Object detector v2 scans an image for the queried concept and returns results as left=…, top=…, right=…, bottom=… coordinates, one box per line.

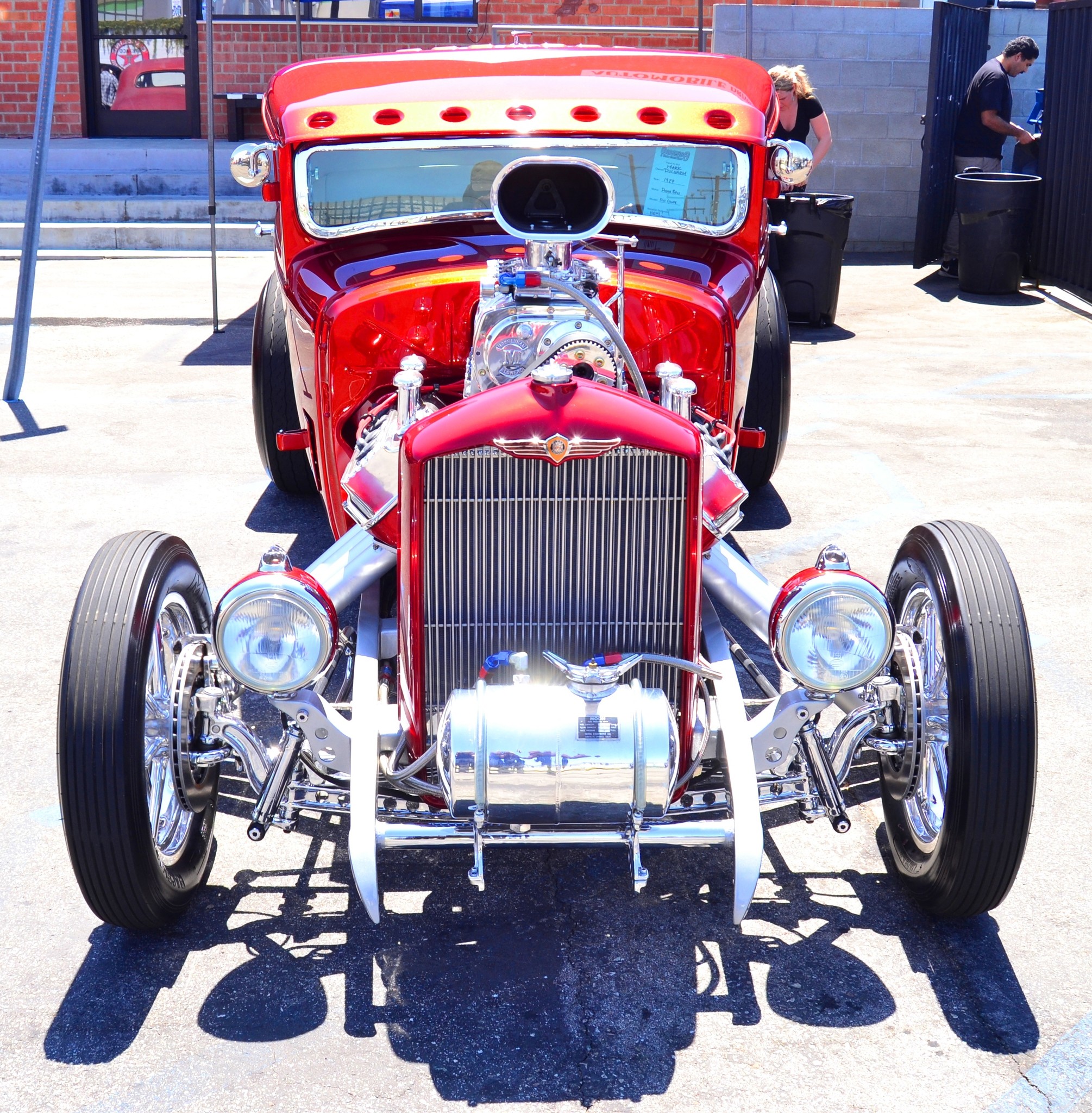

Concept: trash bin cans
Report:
left=954, top=166, right=1042, bottom=296
left=767, top=191, right=853, bottom=330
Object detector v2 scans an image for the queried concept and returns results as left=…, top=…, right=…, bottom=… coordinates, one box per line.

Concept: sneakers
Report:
left=938, top=263, right=959, bottom=278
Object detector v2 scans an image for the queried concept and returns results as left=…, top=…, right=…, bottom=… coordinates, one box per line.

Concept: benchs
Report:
left=213, top=93, right=264, bottom=143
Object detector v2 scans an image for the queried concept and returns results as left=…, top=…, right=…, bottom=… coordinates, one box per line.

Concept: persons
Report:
left=768, top=63, right=832, bottom=194
left=938, top=36, right=1039, bottom=279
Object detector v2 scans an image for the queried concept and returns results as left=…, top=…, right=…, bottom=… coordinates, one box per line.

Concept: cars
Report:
left=56, top=25, right=1039, bottom=938
